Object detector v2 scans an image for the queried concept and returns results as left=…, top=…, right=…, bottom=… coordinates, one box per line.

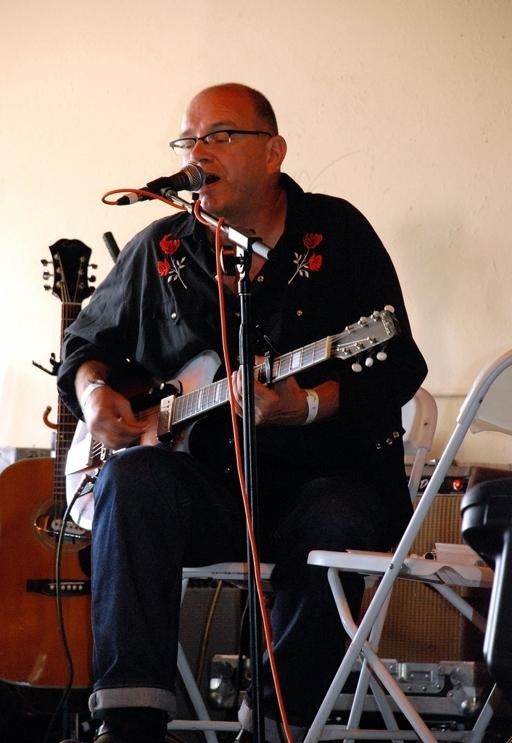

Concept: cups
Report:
left=169, top=129, right=272, bottom=155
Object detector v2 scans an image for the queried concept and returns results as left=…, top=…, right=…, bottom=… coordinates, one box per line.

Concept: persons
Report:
left=59, top=83, right=426, bottom=742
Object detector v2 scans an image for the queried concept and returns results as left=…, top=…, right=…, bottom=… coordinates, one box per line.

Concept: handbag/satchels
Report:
left=304, top=389, right=318, bottom=424
left=79, top=379, right=106, bottom=411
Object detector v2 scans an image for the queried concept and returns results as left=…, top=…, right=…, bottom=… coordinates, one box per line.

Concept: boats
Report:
left=358, top=464, right=472, bottom=664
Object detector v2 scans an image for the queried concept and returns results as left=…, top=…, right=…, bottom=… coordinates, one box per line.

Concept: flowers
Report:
left=117, top=164, right=204, bottom=206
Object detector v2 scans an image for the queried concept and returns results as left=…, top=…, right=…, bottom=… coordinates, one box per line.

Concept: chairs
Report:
left=165, top=352, right=512, bottom=741
